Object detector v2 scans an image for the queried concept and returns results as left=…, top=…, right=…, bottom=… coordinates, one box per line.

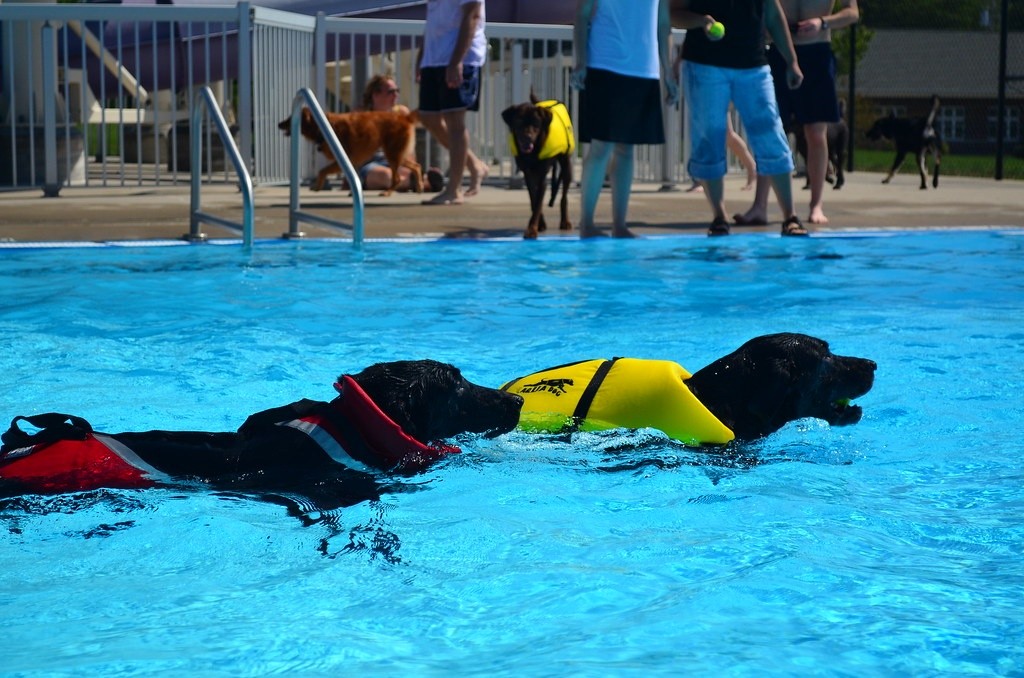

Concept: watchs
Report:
left=818, top=16, right=828, bottom=31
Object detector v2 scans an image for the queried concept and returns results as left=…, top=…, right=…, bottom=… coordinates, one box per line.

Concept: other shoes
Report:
left=410, top=167, right=444, bottom=192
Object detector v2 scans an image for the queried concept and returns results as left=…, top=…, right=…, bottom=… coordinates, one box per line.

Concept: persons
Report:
left=667, top=1, right=808, bottom=237
left=412, top=1, right=492, bottom=206
left=567, top=1, right=681, bottom=240
left=731, top=1, right=860, bottom=225
left=347, top=72, right=445, bottom=194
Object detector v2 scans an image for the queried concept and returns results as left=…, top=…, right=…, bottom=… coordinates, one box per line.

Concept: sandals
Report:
left=780, top=216, right=809, bottom=237
left=709, top=217, right=730, bottom=236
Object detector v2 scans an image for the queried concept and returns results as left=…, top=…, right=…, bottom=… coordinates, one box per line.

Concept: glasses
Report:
left=385, top=87, right=400, bottom=94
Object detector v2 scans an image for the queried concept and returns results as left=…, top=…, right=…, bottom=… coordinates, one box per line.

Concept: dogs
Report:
left=278, top=107, right=429, bottom=197
left=781, top=98, right=850, bottom=190
left=501, top=83, right=575, bottom=240
left=679, top=332, right=877, bottom=446
left=96, top=359, right=525, bottom=491
left=866, top=92, right=944, bottom=190
